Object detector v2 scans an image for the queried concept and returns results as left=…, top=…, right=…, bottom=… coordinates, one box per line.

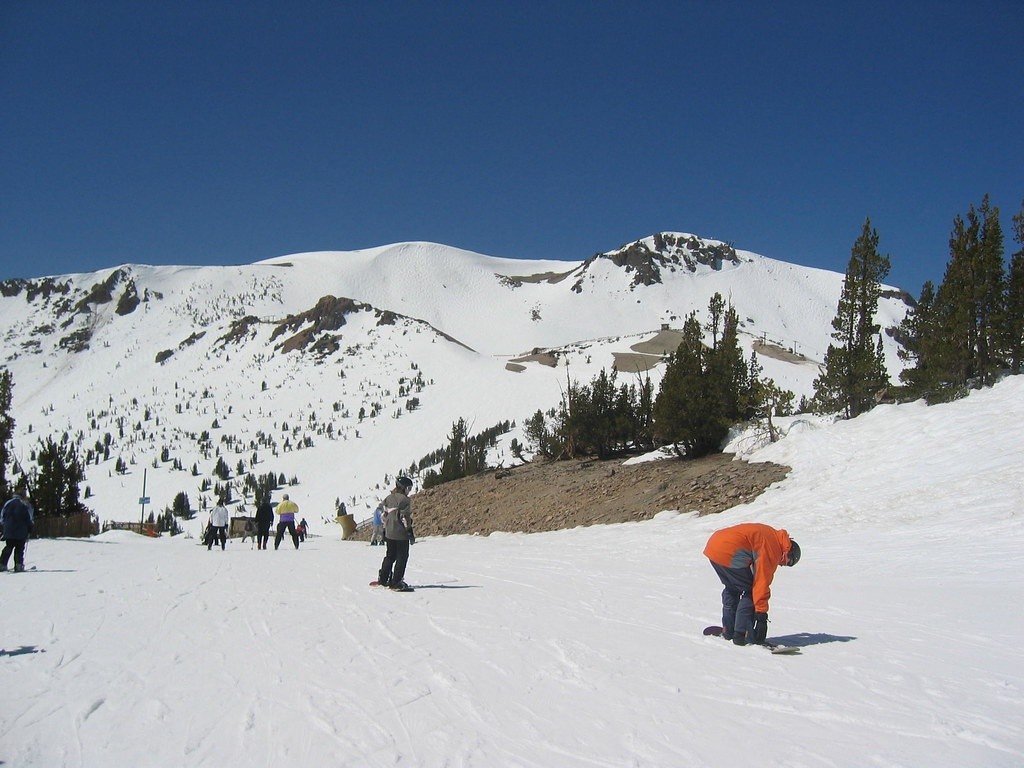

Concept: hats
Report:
left=283, top=494, right=289, bottom=499
left=787, top=540, right=801, bottom=567
left=396, top=477, right=413, bottom=490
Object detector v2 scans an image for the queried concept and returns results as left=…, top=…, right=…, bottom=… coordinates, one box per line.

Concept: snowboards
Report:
left=369, top=581, right=414, bottom=592
left=703, top=626, right=800, bottom=654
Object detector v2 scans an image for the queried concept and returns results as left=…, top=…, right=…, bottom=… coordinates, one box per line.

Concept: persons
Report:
left=257, top=497, right=274, bottom=550
left=378, top=477, right=415, bottom=589
left=703, top=523, right=801, bottom=645
left=208, top=499, right=228, bottom=550
left=370, top=504, right=385, bottom=545
left=0, top=486, right=34, bottom=572
left=201, top=509, right=309, bottom=546
left=274, top=494, right=299, bottom=550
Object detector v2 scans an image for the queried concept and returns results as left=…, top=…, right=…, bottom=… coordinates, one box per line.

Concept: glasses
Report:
left=406, top=486, right=412, bottom=490
left=787, top=559, right=791, bottom=566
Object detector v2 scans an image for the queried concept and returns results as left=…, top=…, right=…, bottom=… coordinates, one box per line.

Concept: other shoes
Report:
left=378, top=581, right=389, bottom=586
left=734, top=632, right=748, bottom=645
left=722, top=627, right=734, bottom=640
left=390, top=579, right=414, bottom=592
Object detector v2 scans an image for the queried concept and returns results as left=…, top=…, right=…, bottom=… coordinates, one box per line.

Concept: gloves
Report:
left=383, top=528, right=387, bottom=543
left=408, top=529, right=415, bottom=545
left=754, top=613, right=769, bottom=641
